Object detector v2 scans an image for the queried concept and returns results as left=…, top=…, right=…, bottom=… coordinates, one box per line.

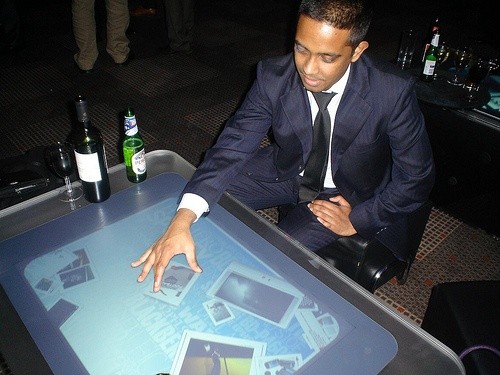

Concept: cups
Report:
left=472, top=57, right=485, bottom=72
left=462, top=79, right=480, bottom=104
left=397, top=28, right=418, bottom=64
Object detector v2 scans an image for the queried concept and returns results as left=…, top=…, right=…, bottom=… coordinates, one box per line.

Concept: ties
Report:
left=298, top=91, right=338, bottom=203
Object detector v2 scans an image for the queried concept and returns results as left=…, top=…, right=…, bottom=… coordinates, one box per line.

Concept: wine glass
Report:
left=48, top=141, right=83, bottom=202
left=447, top=47, right=473, bottom=85
left=433, top=42, right=450, bottom=80
left=487, top=58, right=499, bottom=75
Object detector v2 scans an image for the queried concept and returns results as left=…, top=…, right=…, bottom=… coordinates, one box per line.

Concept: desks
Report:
left=0, top=150, right=465, bottom=375
left=404, top=57, right=491, bottom=108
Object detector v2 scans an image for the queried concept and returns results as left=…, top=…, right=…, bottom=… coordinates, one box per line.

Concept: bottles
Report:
left=69, top=95, right=111, bottom=203
left=421, top=29, right=441, bottom=82
left=122, top=107, right=147, bottom=183
left=420, top=16, right=441, bottom=64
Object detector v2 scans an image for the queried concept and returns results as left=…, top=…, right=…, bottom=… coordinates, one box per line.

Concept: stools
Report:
left=278, top=202, right=434, bottom=295
left=421, top=280, right=500, bottom=375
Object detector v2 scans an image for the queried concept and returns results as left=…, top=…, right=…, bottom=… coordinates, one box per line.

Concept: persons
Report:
left=164, top=0, right=194, bottom=56
left=71, top=0, right=131, bottom=74
left=131, top=0, right=435, bottom=294
